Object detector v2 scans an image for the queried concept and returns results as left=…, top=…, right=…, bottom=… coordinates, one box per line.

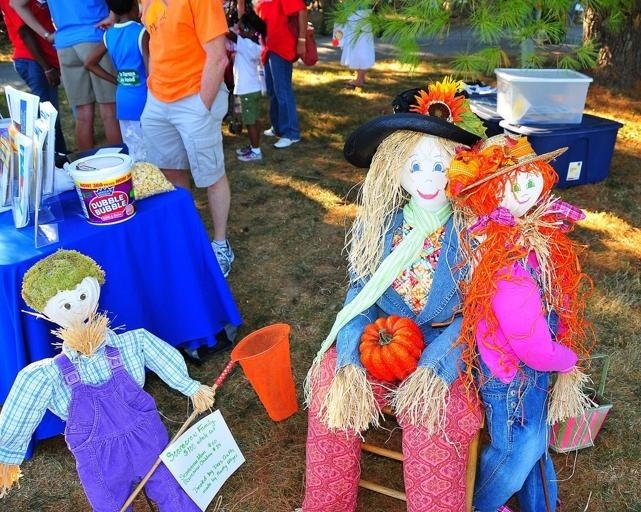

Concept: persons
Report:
left=300, top=85, right=483, bottom=512
left=1, top=248, right=215, bottom=512
left=446, top=132, right=577, bottom=512
left=0, top=0, right=375, bottom=278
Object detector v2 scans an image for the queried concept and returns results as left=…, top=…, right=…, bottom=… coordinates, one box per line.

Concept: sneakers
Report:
left=273, top=136, right=303, bottom=149
left=235, top=144, right=256, bottom=156
left=263, top=126, right=277, bottom=136
left=237, top=152, right=263, bottom=162
left=344, top=79, right=366, bottom=88
left=210, top=239, right=238, bottom=278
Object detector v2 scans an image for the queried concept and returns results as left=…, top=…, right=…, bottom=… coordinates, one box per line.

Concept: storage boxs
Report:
left=500, top=112, right=623, bottom=188
left=470, top=101, right=503, bottom=138
left=496, top=68, right=593, bottom=124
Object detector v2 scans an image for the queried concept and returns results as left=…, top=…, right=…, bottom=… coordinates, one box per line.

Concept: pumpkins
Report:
left=359, top=315, right=424, bottom=383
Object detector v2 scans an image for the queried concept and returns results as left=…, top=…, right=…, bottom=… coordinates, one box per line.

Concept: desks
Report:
left=0, top=147, right=243, bottom=460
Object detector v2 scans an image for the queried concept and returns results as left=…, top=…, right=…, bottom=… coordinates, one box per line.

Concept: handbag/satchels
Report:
left=286, top=12, right=319, bottom=66
